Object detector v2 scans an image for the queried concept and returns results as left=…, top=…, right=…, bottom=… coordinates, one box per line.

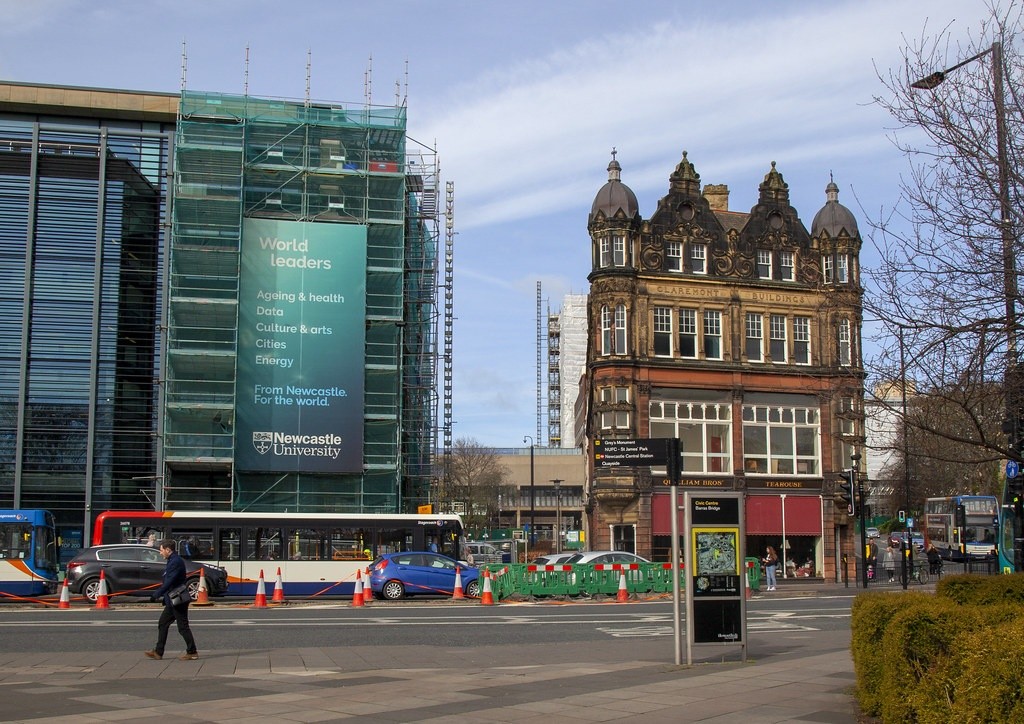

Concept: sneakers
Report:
left=769, top=586, right=776, bottom=591
left=767, top=586, right=770, bottom=591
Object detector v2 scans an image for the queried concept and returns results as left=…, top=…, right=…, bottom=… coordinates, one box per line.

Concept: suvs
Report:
left=65, top=543, right=228, bottom=604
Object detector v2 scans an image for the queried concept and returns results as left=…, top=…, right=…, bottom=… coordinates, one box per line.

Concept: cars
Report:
left=888, top=531, right=924, bottom=551
left=865, top=527, right=880, bottom=538
left=367, top=551, right=667, bottom=599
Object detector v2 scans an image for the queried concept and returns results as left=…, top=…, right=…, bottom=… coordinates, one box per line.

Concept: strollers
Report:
left=932, top=556, right=945, bottom=573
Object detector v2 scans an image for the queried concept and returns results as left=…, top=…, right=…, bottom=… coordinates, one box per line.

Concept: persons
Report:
left=883, top=546, right=895, bottom=582
left=912, top=542, right=921, bottom=578
left=143, top=534, right=157, bottom=562
left=762, top=546, right=777, bottom=591
left=146, top=540, right=199, bottom=660
left=927, top=543, right=939, bottom=574
left=758, top=556, right=814, bottom=577
left=429, top=557, right=449, bottom=568
left=867, top=538, right=878, bottom=581
left=430, top=537, right=449, bottom=555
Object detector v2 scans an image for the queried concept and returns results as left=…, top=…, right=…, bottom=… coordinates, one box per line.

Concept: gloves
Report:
left=150, top=597, right=155, bottom=602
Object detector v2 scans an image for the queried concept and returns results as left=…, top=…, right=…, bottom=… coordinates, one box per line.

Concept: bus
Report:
left=998, top=469, right=1014, bottom=574
left=923, top=495, right=1002, bottom=564
left=0, top=509, right=468, bottom=598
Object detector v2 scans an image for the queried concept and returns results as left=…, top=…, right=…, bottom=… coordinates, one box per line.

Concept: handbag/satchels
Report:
left=168, top=584, right=192, bottom=608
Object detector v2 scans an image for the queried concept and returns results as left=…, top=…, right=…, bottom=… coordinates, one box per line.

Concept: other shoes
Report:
left=888, top=579, right=891, bottom=583
left=145, top=647, right=162, bottom=660
left=891, top=577, right=895, bottom=582
left=178, top=651, right=199, bottom=660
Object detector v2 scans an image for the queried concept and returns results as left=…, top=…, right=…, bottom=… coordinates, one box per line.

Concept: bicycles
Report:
left=899, top=558, right=929, bottom=585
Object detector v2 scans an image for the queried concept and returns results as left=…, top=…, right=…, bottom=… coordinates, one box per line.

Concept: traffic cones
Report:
left=450, top=564, right=501, bottom=606
left=249, top=567, right=287, bottom=609
left=614, top=566, right=631, bottom=603
left=190, top=568, right=215, bottom=606
left=349, top=566, right=375, bottom=607
left=57, top=568, right=115, bottom=611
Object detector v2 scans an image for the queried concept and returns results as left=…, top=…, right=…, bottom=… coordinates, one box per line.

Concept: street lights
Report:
left=910, top=40, right=1024, bottom=572
left=550, top=479, right=565, bottom=553
left=523, top=436, right=534, bottom=551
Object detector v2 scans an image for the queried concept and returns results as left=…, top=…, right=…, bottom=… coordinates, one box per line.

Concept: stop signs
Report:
left=840, top=469, right=854, bottom=517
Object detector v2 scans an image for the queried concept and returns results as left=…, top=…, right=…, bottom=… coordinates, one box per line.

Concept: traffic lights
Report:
left=899, top=510, right=905, bottom=522
